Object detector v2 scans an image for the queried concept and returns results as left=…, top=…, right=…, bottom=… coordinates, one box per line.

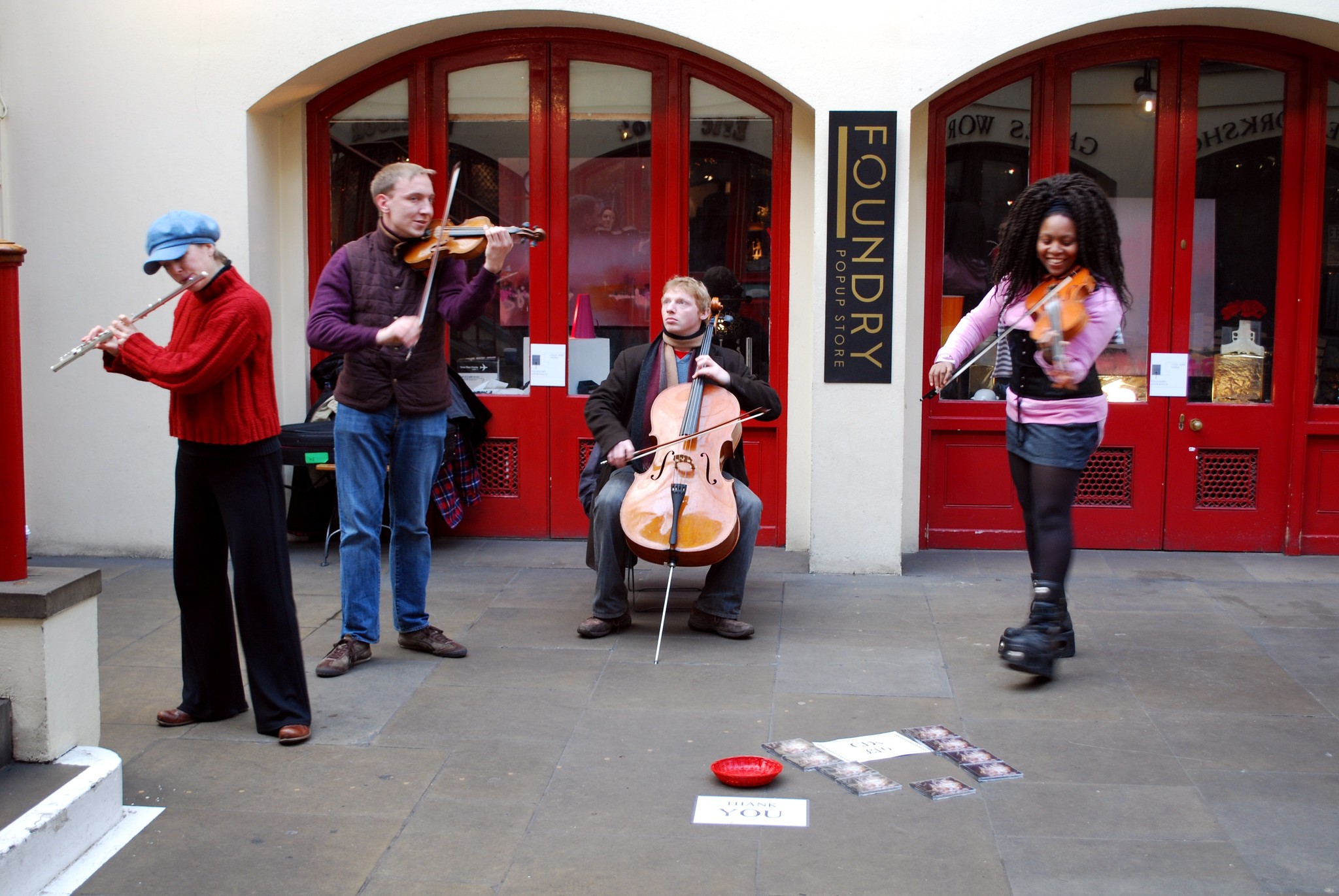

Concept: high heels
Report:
left=1005, top=579, right=1063, bottom=677
left=997, top=608, right=1076, bottom=657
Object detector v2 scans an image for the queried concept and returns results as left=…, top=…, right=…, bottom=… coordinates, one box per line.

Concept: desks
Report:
left=315, top=464, right=391, bottom=567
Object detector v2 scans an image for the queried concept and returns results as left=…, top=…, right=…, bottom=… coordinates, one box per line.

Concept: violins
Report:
left=1025, top=266, right=1097, bottom=390
left=403, top=215, right=546, bottom=271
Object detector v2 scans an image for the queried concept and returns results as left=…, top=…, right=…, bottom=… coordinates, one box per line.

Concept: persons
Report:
left=568, top=193, right=770, bottom=381
left=930, top=174, right=1133, bottom=680
left=306, top=163, right=514, bottom=676
left=577, top=272, right=783, bottom=641
left=80, top=211, right=312, bottom=745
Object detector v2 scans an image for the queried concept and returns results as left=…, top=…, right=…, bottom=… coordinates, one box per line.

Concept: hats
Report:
left=144, top=211, right=219, bottom=275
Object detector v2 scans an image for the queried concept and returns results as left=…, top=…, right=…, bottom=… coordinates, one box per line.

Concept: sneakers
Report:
left=578, top=611, right=632, bottom=637
left=397, top=624, right=467, bottom=658
left=687, top=600, right=755, bottom=637
left=316, top=634, right=372, bottom=676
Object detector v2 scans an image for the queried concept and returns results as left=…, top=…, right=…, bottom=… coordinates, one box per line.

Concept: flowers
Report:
left=1220, top=297, right=1267, bottom=326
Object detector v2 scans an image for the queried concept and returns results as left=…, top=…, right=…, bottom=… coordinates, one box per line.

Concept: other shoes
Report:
left=278, top=723, right=311, bottom=743
left=157, top=708, right=192, bottom=727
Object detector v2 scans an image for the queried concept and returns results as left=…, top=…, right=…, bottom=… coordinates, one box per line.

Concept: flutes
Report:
left=50, top=270, right=209, bottom=373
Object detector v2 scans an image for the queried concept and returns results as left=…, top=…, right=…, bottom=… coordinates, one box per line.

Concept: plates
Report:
left=711, top=756, right=783, bottom=786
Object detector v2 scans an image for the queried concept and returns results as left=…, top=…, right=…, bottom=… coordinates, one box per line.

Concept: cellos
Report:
left=619, top=296, right=742, bottom=664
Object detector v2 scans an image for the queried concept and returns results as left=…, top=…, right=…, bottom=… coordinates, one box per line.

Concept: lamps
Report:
left=1130, top=59, right=1157, bottom=121
